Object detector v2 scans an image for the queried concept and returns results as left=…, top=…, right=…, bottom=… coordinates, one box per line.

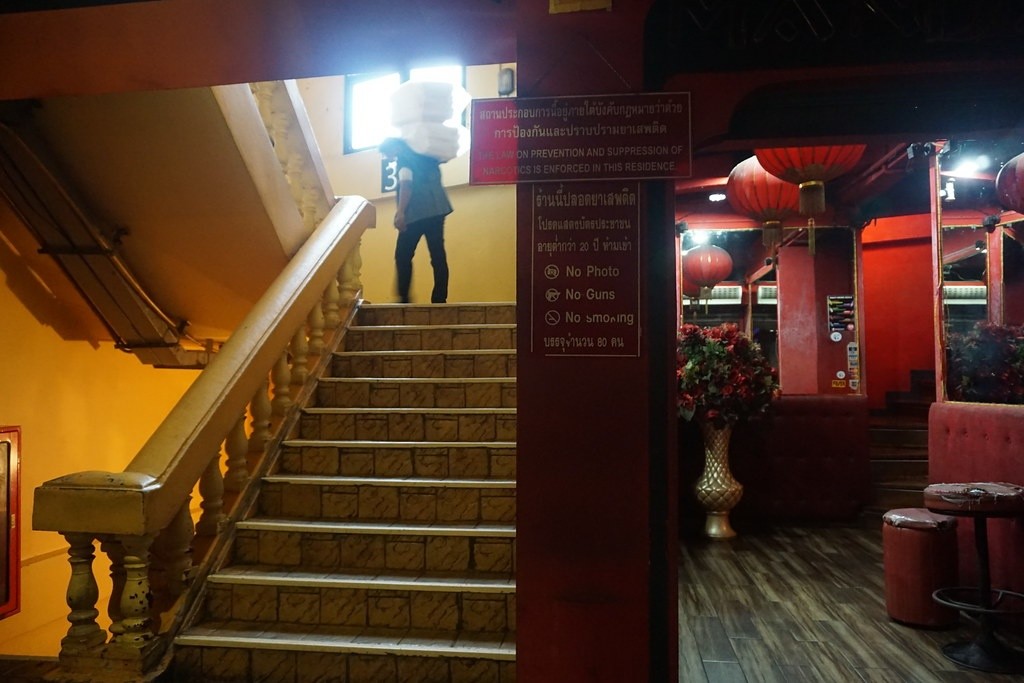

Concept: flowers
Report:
left=674, top=318, right=783, bottom=426
left=943, top=318, right=1024, bottom=401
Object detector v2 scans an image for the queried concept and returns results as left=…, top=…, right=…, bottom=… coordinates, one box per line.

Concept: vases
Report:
left=694, top=423, right=744, bottom=542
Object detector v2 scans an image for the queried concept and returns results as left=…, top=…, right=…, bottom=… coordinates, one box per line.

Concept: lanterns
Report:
left=724, top=143, right=866, bottom=272
left=994, top=152, right=1024, bottom=213
left=682, top=247, right=735, bottom=320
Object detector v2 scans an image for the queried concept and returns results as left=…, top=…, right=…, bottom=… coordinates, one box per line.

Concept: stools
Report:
left=882, top=507, right=963, bottom=629
left=922, top=481, right=1023, bottom=673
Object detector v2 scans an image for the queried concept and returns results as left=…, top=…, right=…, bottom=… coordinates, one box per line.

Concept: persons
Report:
left=378, top=137, right=450, bottom=306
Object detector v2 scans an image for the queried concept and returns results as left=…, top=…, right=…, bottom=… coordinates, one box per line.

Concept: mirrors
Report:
left=935, top=129, right=1024, bottom=407
